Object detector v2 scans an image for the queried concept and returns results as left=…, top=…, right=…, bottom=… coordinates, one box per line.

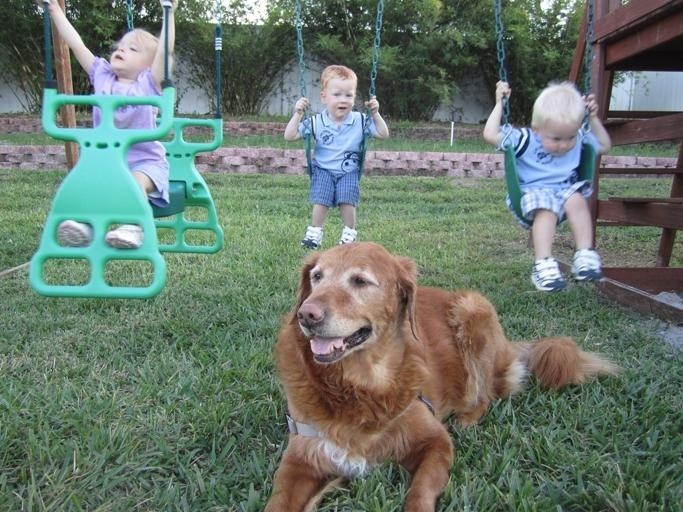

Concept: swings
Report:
left=295, top=0, right=384, bottom=180
left=494, top=0, right=597, bottom=225
left=28, top=0, right=224, bottom=299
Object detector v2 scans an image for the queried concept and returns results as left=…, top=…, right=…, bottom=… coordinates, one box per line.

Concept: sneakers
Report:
left=338, top=226, right=358, bottom=245
left=571, top=248, right=602, bottom=283
left=56, top=218, right=93, bottom=248
left=300, top=226, right=324, bottom=250
left=531, top=255, right=567, bottom=292
left=105, top=223, right=144, bottom=248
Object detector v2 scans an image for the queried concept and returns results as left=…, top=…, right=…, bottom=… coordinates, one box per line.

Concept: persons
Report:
left=41, top=0, right=179, bottom=250
left=482, top=80, right=612, bottom=293
left=284, top=65, right=389, bottom=248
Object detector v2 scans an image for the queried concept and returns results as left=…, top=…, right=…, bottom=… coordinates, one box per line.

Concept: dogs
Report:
left=262, top=240, right=624, bottom=512
